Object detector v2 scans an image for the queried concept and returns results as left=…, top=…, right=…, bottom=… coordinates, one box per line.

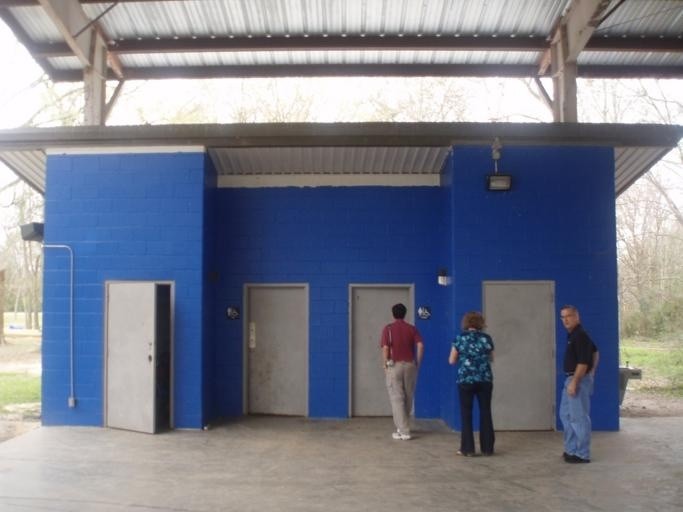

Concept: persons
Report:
left=381, top=303, right=423, bottom=439
left=559, top=305, right=600, bottom=463
left=449, top=312, right=494, bottom=456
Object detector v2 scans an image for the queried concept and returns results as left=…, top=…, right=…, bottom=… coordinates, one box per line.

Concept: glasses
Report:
left=560, top=313, right=574, bottom=320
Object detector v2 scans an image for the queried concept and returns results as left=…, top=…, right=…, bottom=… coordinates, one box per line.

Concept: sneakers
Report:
left=392, top=432, right=412, bottom=440
left=457, top=448, right=474, bottom=458
left=564, top=451, right=589, bottom=463
